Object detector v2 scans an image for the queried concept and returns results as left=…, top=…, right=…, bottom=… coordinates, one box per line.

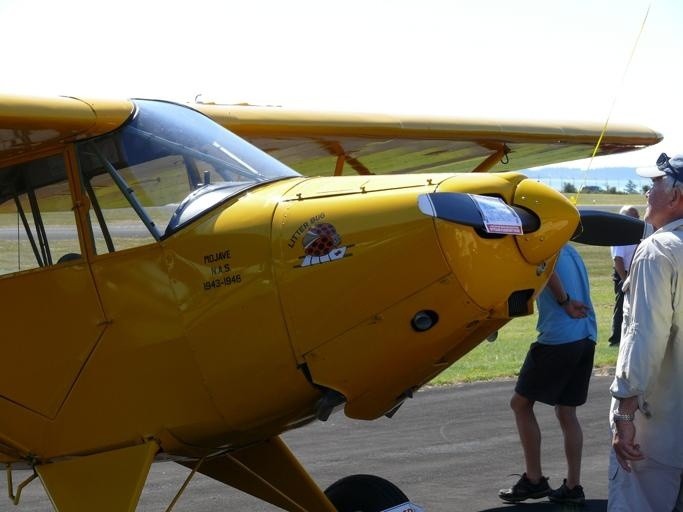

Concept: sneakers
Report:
left=548, top=477, right=588, bottom=507
left=498, top=472, right=553, bottom=505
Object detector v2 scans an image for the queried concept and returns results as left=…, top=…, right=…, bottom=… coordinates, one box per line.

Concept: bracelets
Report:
left=612, top=413, right=634, bottom=422
left=557, top=293, right=570, bottom=305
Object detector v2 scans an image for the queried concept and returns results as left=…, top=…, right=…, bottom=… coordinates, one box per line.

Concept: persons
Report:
left=325, top=473, right=422, bottom=512
left=606, top=157, right=683, bottom=512
left=608, top=204, right=641, bottom=344
left=496, top=239, right=597, bottom=511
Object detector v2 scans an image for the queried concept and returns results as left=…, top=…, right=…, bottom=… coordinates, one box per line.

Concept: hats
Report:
left=635, top=153, right=683, bottom=185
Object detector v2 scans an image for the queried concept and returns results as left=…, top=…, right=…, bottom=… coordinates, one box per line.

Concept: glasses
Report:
left=656, top=152, right=678, bottom=188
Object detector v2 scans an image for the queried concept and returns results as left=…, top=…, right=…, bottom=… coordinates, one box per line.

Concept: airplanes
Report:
left=0, top=93, right=663, bottom=512
